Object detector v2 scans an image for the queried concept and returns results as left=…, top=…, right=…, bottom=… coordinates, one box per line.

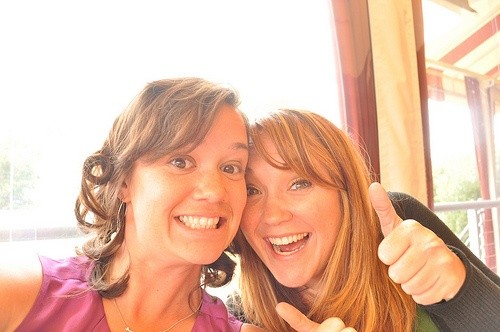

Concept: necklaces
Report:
left=98, top=266, right=212, bottom=332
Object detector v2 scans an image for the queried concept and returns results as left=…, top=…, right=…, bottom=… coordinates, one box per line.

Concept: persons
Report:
left=0, top=79, right=271, bottom=332
left=226, top=109, right=499, bottom=332
